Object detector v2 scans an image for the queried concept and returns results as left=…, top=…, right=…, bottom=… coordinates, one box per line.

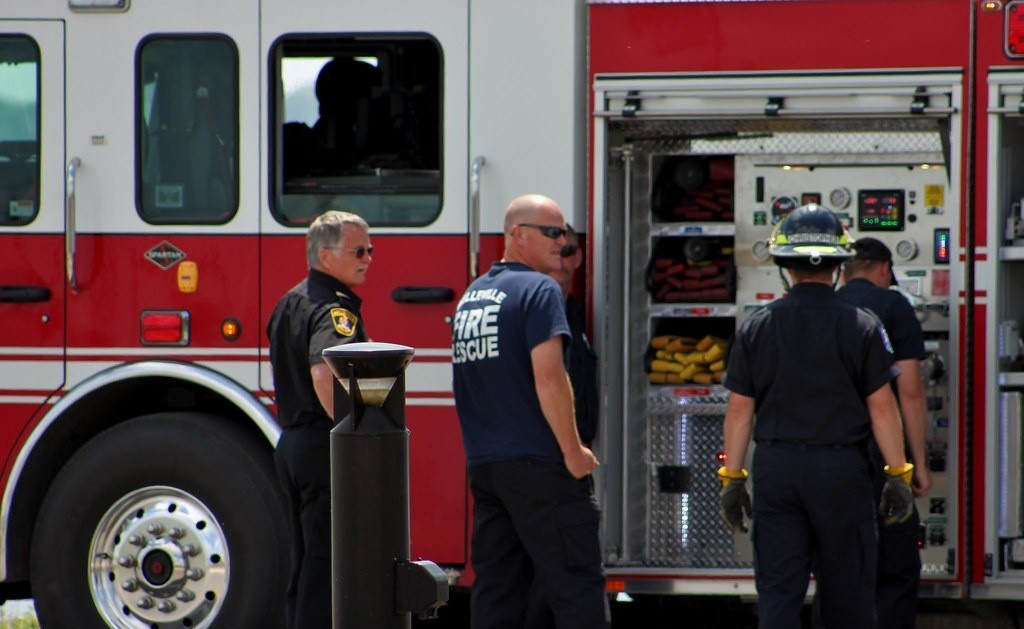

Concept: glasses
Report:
left=324, top=246, right=374, bottom=259
left=560, top=245, right=580, bottom=257
left=511, top=223, right=567, bottom=239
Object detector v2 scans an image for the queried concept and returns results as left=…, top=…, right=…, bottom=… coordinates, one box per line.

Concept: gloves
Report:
left=718, top=466, right=754, bottom=534
left=879, top=463, right=914, bottom=525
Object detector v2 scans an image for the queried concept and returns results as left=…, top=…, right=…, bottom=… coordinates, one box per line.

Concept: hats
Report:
left=850, top=238, right=899, bottom=285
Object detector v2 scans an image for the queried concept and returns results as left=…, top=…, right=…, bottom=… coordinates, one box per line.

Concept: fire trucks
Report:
left=0, top=0, right=1024, bottom=629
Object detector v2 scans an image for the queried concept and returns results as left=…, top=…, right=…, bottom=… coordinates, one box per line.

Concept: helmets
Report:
left=768, top=202, right=858, bottom=258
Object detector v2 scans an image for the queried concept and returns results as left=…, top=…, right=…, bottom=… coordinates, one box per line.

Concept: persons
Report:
left=451, top=194, right=611, bottom=629
left=266, top=209, right=376, bottom=629
left=716, top=204, right=915, bottom=628
left=525, top=224, right=598, bottom=627
left=835, top=237, right=930, bottom=628
